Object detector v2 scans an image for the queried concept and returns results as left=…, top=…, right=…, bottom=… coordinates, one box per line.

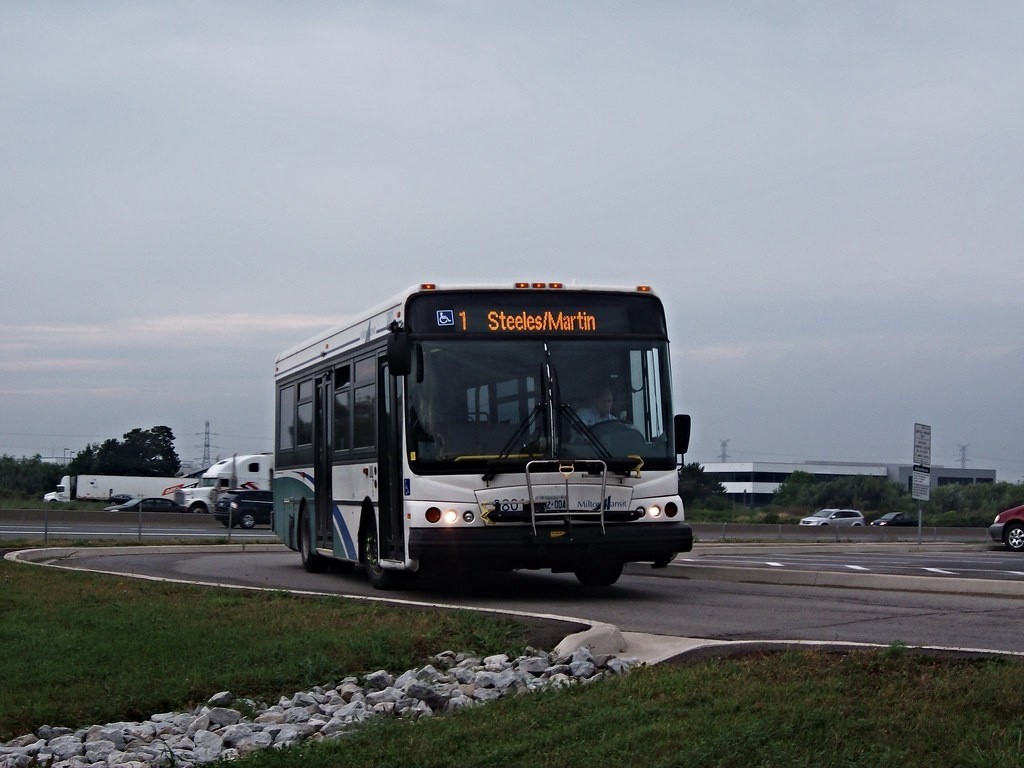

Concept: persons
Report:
left=569, top=387, right=617, bottom=445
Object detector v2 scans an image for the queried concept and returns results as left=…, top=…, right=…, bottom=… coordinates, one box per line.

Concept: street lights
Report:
left=63, top=447, right=69, bottom=464
left=69, top=450, right=76, bottom=463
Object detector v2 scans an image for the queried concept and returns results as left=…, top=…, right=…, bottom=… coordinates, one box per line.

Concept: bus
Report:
left=271, top=281, right=694, bottom=591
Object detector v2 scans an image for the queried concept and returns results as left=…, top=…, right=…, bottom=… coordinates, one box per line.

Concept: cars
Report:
left=102, top=496, right=188, bottom=512
left=105, top=493, right=133, bottom=505
left=988, top=505, right=1024, bottom=552
left=870, top=512, right=927, bottom=526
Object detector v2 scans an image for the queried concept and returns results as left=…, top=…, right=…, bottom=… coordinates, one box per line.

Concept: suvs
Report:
left=216, top=490, right=273, bottom=529
left=799, top=509, right=867, bottom=527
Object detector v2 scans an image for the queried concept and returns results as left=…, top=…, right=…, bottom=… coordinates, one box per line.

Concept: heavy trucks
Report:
left=43, top=475, right=201, bottom=503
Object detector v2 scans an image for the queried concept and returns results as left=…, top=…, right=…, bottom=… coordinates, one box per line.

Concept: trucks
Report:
left=174, top=452, right=274, bottom=515
